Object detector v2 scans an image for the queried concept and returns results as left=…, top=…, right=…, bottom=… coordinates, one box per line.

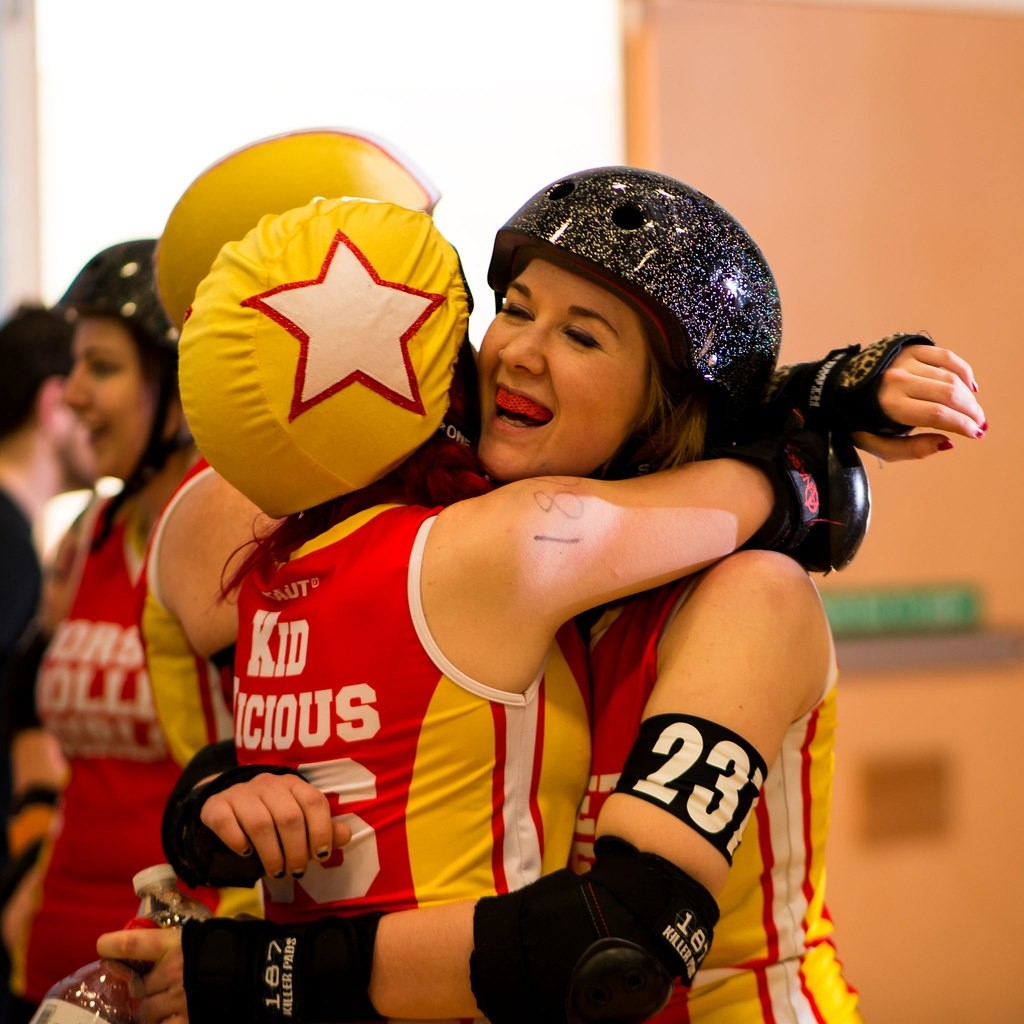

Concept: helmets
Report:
left=156, top=127, right=442, bottom=336
left=178, top=196, right=469, bottom=520
left=487, top=168, right=781, bottom=429
left=58, top=238, right=181, bottom=399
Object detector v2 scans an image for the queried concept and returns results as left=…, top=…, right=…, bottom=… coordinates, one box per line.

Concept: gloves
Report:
left=713, top=398, right=870, bottom=574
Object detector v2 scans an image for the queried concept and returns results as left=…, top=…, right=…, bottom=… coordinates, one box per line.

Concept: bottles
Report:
left=133, top=864, right=213, bottom=1022
left=29, top=917, right=162, bottom=1024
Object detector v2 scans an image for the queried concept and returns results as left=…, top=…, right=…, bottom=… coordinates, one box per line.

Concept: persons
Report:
left=1, top=127, right=441, bottom=1024
left=94, top=168, right=869, bottom=1024
left=176, top=198, right=989, bottom=1024
